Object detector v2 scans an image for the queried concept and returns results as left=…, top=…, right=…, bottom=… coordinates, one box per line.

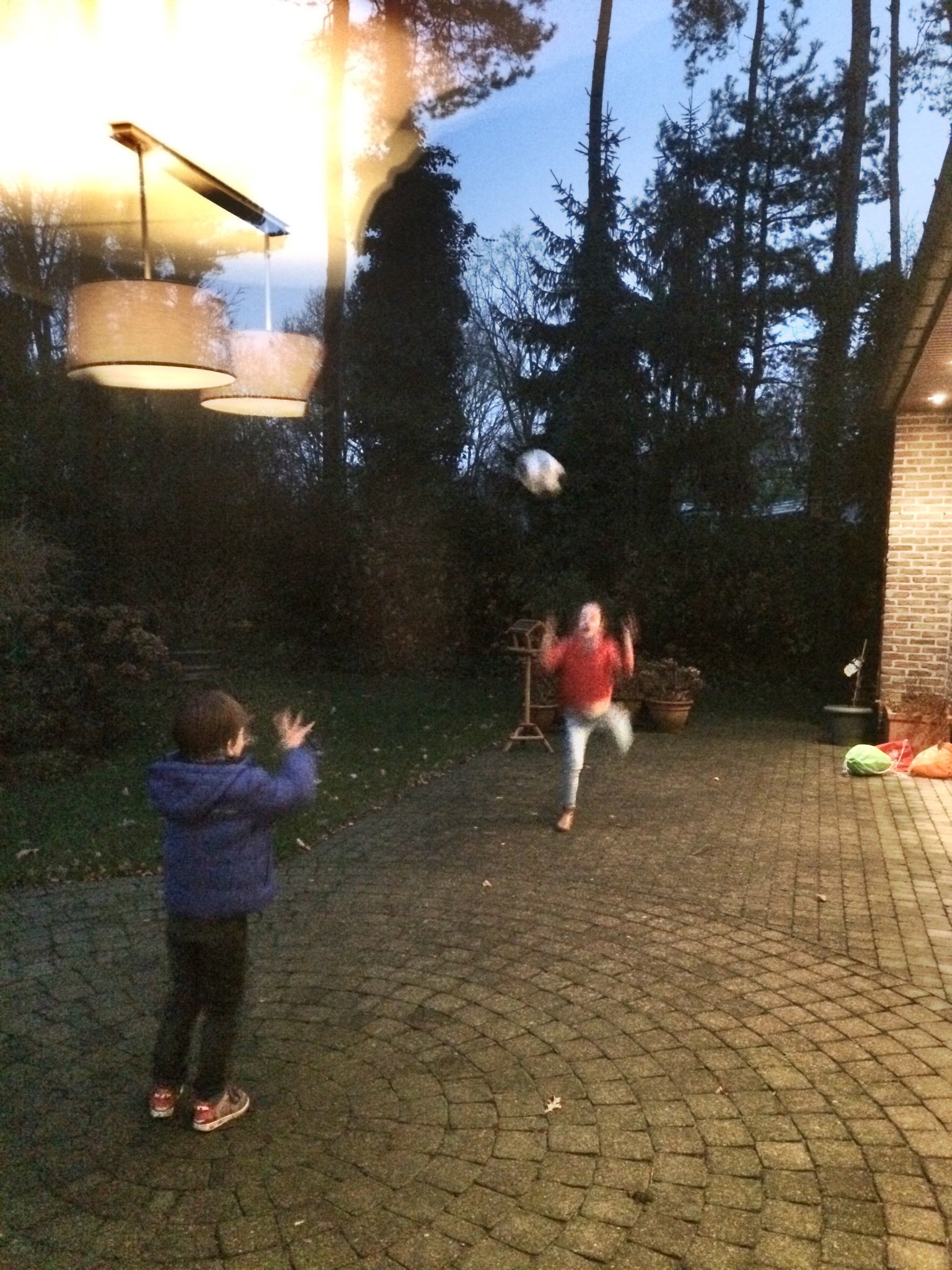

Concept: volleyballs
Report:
left=514, top=449, right=567, bottom=499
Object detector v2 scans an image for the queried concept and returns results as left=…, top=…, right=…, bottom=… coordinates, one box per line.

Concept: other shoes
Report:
left=556, top=808, right=574, bottom=830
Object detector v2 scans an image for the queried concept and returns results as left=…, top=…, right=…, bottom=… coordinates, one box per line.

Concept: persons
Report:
left=146, top=691, right=323, bottom=1133
left=539, top=600, right=636, bottom=832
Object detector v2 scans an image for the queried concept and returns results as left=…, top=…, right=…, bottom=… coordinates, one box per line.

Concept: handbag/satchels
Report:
left=842, top=744, right=892, bottom=777
left=908, top=742, right=952, bottom=778
left=874, top=739, right=913, bottom=771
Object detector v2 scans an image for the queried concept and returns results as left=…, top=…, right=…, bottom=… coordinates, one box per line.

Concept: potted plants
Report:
left=522, top=683, right=560, bottom=727
left=821, top=639, right=873, bottom=745
left=884, top=691, right=952, bottom=749
left=612, top=654, right=702, bottom=732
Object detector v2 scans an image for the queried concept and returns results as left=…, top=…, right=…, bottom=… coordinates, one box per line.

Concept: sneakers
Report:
left=150, top=1082, right=184, bottom=1117
left=194, top=1086, right=250, bottom=1132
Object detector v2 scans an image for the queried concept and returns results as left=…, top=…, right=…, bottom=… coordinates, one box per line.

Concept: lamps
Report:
left=64, top=123, right=325, bottom=418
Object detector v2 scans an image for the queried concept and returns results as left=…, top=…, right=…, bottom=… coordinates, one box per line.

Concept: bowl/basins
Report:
left=823, top=704, right=873, bottom=746
